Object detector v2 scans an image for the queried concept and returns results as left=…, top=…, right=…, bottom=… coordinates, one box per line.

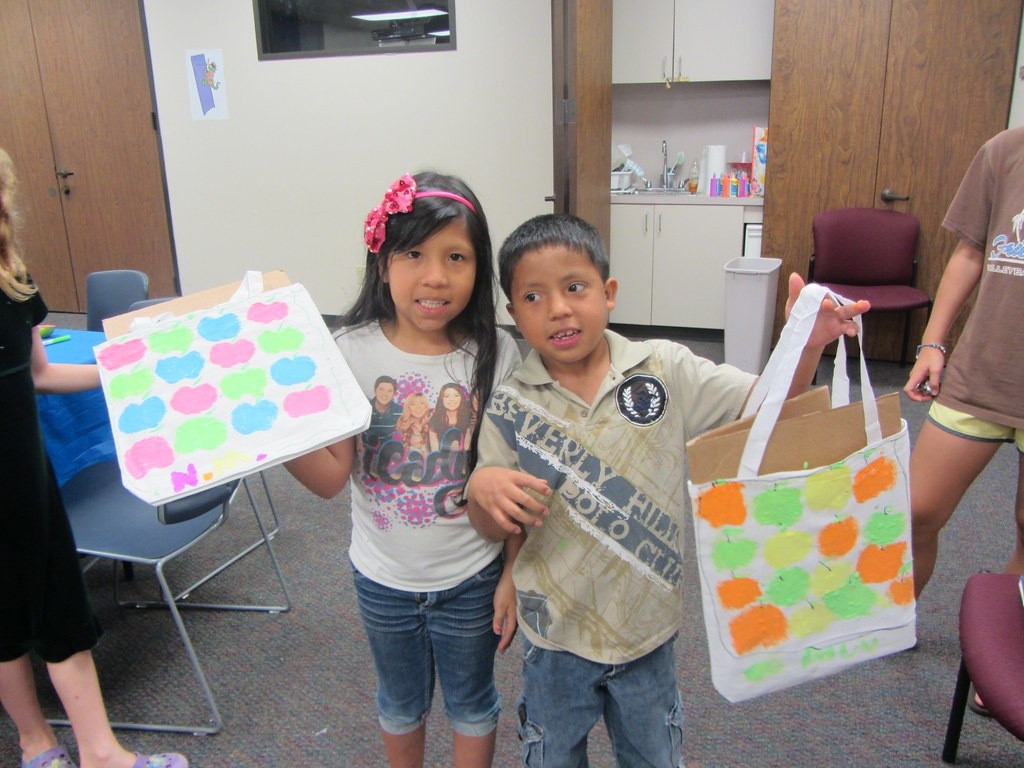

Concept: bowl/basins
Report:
left=610, top=168, right=632, bottom=190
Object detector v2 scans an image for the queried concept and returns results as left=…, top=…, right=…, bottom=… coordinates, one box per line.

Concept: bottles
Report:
left=710, top=170, right=750, bottom=197
left=689, top=159, right=699, bottom=194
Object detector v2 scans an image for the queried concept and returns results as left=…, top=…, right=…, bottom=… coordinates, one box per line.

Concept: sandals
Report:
left=967, top=680, right=992, bottom=718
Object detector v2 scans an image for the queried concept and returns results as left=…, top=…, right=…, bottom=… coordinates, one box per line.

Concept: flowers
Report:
left=363, top=172, right=417, bottom=254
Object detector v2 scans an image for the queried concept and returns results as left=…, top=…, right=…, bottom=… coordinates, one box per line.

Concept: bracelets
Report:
left=914, top=343, right=947, bottom=358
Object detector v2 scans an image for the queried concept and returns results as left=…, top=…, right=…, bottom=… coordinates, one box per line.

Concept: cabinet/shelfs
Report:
left=607, top=201, right=745, bottom=331
left=612, top=0, right=775, bottom=89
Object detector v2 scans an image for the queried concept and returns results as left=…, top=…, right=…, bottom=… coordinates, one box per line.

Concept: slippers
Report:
left=131, top=751, right=189, bottom=768
left=21, top=744, right=79, bottom=768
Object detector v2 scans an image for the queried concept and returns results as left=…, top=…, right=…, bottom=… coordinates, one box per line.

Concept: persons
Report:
left=279, top=172, right=528, bottom=768
left=0, top=149, right=189, bottom=768
left=462, top=212, right=871, bottom=767
left=902, top=127, right=1024, bottom=718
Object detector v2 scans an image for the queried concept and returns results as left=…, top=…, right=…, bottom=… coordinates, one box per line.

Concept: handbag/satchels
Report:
left=684, top=280, right=920, bottom=704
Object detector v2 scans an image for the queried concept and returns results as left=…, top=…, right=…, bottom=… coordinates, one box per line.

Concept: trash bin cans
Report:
left=723, top=256, right=782, bottom=376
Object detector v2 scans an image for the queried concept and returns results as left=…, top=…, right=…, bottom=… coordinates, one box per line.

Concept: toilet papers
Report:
left=697, top=145, right=727, bottom=196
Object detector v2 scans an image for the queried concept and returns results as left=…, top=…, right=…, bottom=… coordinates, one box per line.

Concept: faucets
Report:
left=661, top=140, right=668, bottom=188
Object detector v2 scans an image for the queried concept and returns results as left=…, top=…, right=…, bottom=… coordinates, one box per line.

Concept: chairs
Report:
left=43, top=269, right=295, bottom=735
left=940, top=568, right=1024, bottom=764
left=805, top=208, right=937, bottom=388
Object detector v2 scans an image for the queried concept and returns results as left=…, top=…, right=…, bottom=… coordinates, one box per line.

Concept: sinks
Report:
left=635, top=188, right=691, bottom=195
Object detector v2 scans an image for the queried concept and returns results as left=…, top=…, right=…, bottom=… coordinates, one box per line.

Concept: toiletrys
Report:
left=710, top=169, right=751, bottom=197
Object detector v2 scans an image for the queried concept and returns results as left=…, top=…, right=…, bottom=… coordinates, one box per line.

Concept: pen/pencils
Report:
left=43, top=335, right=73, bottom=346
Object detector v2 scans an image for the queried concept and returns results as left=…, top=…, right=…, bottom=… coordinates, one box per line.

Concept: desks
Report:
left=28, top=328, right=135, bottom=581
